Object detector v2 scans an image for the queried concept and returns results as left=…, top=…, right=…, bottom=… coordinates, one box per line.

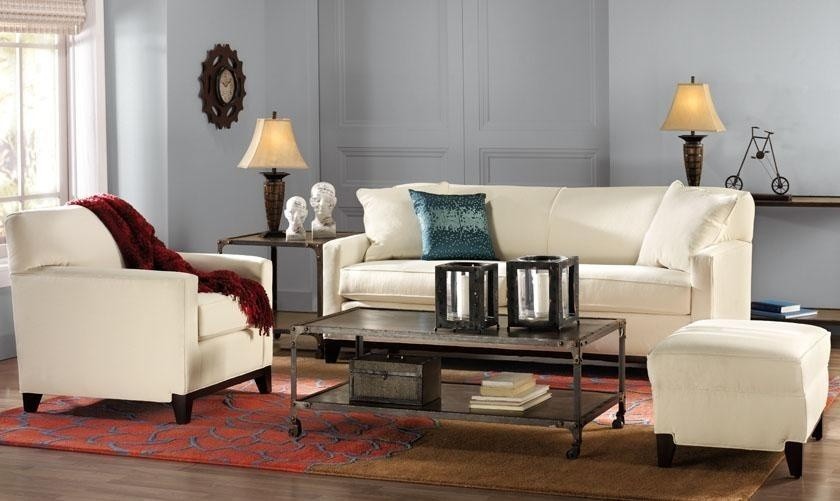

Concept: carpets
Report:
left=0, top=356, right=840, bottom=501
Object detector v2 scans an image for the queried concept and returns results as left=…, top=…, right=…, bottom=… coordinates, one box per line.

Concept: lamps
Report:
left=660, top=76, right=727, bottom=186
left=434, top=262, right=500, bottom=333
left=507, top=254, right=581, bottom=337
left=237, top=111, right=309, bottom=238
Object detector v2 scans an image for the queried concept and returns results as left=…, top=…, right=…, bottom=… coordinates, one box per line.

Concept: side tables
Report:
left=216, top=230, right=365, bottom=359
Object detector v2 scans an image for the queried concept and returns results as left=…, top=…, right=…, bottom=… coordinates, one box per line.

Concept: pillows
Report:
left=408, top=188, right=499, bottom=260
left=636, top=180, right=739, bottom=274
left=356, top=181, right=449, bottom=262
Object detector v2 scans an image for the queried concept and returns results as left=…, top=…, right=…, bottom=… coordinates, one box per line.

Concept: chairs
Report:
left=4, top=202, right=273, bottom=424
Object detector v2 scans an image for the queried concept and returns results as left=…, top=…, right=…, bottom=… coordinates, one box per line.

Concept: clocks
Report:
left=197, top=44, right=246, bottom=129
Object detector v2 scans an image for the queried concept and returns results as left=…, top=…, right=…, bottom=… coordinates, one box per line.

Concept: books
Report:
left=469, top=372, right=552, bottom=411
left=751, top=298, right=819, bottom=319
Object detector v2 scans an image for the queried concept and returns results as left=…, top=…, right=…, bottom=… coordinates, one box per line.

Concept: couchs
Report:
left=322, top=181, right=755, bottom=379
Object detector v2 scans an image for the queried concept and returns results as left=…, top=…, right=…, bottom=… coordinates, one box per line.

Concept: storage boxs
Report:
left=348, top=353, right=442, bottom=410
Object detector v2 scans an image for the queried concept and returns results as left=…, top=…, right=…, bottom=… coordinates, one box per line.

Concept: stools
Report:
left=646, top=319, right=832, bottom=479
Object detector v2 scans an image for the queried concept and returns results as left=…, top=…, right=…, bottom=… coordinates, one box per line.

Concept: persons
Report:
left=309, top=182, right=337, bottom=225
left=284, top=196, right=309, bottom=232
